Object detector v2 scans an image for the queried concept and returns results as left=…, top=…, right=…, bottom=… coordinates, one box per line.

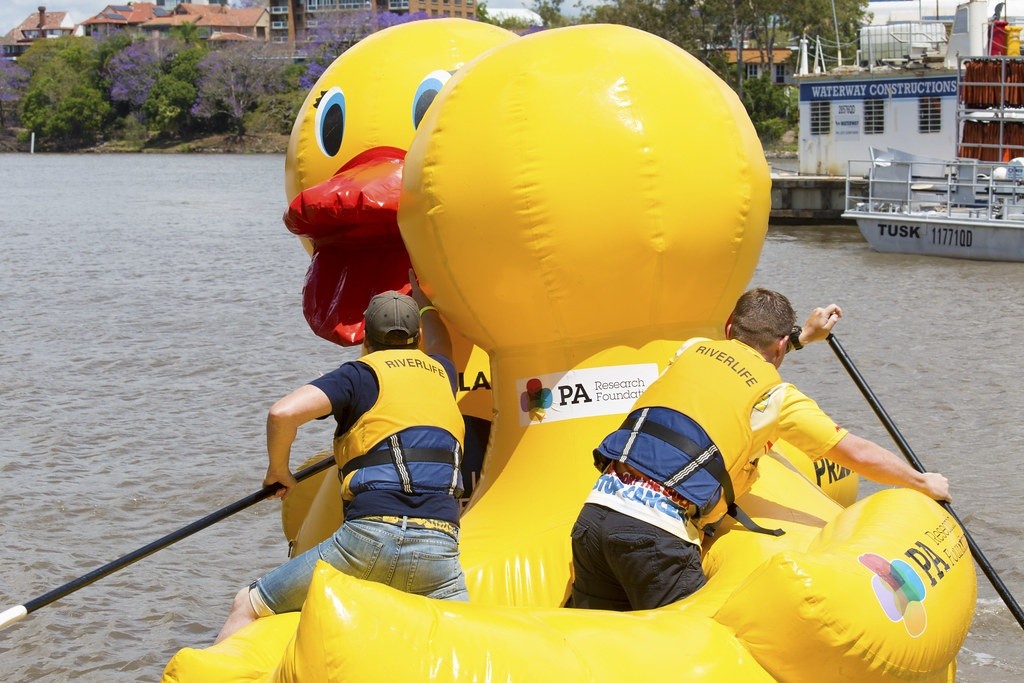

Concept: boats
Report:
left=839, top=160, right=1024, bottom=264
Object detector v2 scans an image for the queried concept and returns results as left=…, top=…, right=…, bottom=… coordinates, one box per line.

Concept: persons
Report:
left=571, top=289, right=952, bottom=609
left=212, top=291, right=469, bottom=652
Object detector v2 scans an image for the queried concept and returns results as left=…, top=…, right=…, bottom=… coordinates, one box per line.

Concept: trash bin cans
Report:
left=988, top=21, right=1008, bottom=55
left=1005, top=26, right=1024, bottom=56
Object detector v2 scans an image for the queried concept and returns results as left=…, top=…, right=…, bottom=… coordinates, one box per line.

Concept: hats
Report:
left=363, top=290, right=420, bottom=346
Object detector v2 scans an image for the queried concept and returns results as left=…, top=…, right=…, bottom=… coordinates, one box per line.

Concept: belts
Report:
left=357, top=514, right=459, bottom=544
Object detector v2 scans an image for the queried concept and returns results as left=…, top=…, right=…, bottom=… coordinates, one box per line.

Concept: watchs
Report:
left=789, top=326, right=804, bottom=351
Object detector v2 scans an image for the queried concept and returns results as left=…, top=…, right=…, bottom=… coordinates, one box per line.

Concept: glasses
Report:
left=778, top=333, right=791, bottom=354
